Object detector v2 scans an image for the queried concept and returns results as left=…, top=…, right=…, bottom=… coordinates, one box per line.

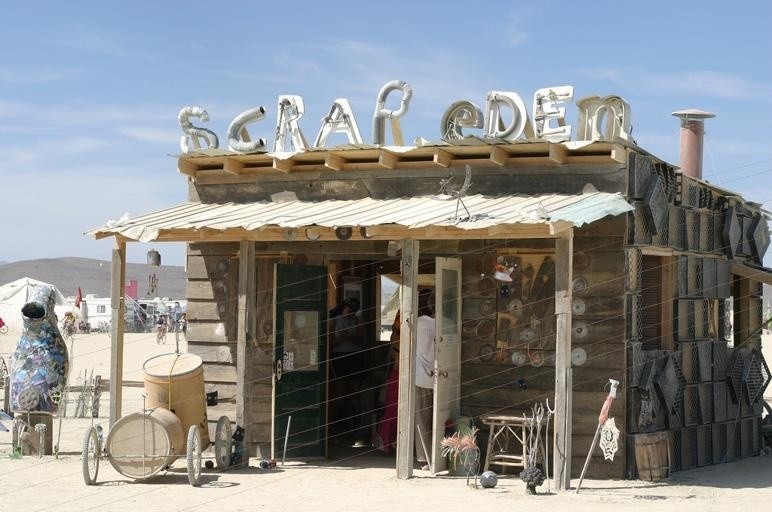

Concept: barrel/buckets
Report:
left=142, top=351, right=210, bottom=458
left=104, top=408, right=185, bottom=479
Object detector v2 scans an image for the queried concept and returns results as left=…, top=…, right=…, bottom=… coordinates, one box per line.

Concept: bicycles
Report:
left=62, top=321, right=78, bottom=340
left=157, top=330, right=168, bottom=345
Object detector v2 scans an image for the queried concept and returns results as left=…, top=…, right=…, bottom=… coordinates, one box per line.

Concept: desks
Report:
left=482, top=415, right=546, bottom=477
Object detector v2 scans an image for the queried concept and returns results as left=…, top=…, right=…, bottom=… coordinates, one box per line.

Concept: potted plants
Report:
left=439, top=426, right=482, bottom=488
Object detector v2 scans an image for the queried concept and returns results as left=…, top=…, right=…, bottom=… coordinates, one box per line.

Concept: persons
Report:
left=414, top=306, right=456, bottom=471
left=329, top=296, right=366, bottom=352
left=370, top=310, right=401, bottom=451
left=156, top=313, right=167, bottom=327
left=178, top=311, right=188, bottom=339
